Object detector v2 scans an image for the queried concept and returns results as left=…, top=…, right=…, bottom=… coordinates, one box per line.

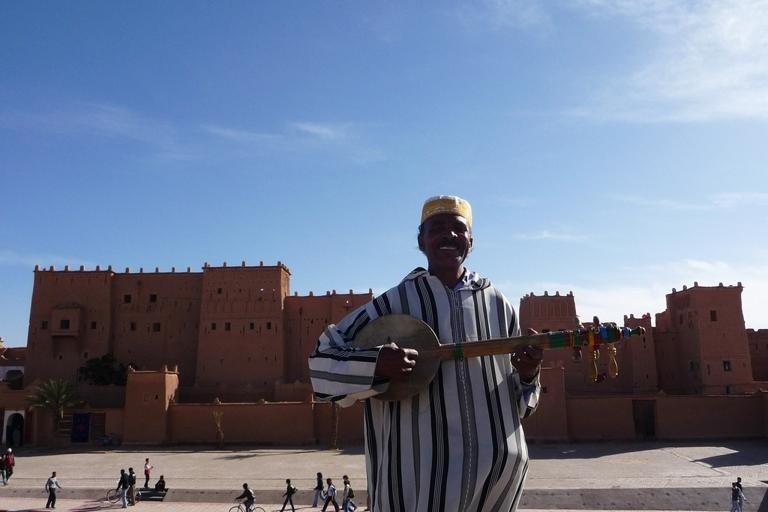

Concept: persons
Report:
left=5, top=447, right=15, bottom=480
left=280, top=472, right=354, bottom=512
left=308, top=195, right=543, bottom=511
left=730, top=483, right=747, bottom=512
left=116, top=458, right=165, bottom=508
left=0, top=451, right=8, bottom=486
left=46, top=471, right=62, bottom=508
left=735, top=477, right=743, bottom=511
left=235, top=483, right=256, bottom=512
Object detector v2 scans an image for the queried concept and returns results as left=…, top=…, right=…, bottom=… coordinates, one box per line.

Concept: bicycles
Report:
left=228, top=497, right=265, bottom=512
left=106, top=482, right=140, bottom=505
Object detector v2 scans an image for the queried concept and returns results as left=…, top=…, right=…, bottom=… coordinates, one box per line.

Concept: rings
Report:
left=516, top=358, right=520, bottom=361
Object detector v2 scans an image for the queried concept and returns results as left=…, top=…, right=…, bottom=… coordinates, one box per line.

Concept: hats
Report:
left=419, top=194, right=473, bottom=229
